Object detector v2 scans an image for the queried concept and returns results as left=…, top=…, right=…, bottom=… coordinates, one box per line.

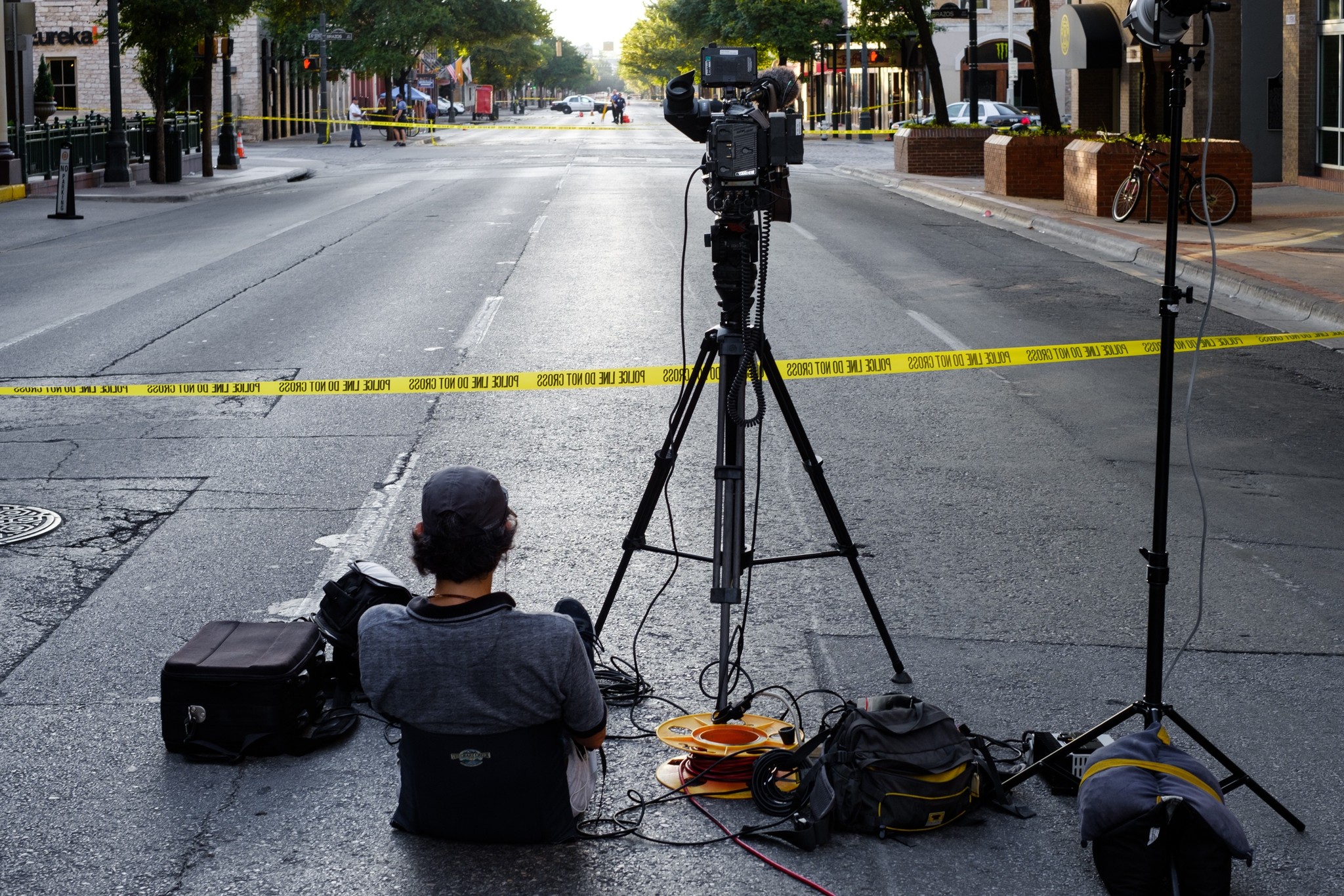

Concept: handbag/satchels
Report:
left=1079, top=717, right=1252, bottom=896
left=788, top=693, right=1005, bottom=840
left=162, top=620, right=360, bottom=761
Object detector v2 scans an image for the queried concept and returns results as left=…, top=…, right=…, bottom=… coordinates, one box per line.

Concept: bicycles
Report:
left=378, top=112, right=420, bottom=138
left=1112, top=131, right=1239, bottom=226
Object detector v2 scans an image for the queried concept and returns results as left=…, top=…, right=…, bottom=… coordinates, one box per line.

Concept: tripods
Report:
left=995, top=44, right=1308, bottom=829
left=594, top=215, right=910, bottom=719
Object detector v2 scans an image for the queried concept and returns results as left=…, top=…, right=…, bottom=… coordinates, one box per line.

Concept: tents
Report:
left=376, top=83, right=431, bottom=132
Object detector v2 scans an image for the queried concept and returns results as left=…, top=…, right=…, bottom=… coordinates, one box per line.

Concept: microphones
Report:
left=741, top=64, right=801, bottom=111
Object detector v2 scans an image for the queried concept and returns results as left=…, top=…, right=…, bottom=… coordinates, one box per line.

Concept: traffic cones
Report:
left=236, top=132, right=248, bottom=158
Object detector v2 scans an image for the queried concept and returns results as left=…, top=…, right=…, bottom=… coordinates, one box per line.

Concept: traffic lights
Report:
left=220, top=36, right=235, bottom=54
left=303, top=57, right=317, bottom=69
left=870, top=49, right=882, bottom=62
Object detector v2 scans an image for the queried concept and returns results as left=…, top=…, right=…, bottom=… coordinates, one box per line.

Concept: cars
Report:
left=550, top=94, right=613, bottom=114
left=437, top=95, right=465, bottom=117
left=889, top=98, right=1042, bottom=141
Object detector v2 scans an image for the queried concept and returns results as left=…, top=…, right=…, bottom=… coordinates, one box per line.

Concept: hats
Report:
left=421, top=465, right=508, bottom=535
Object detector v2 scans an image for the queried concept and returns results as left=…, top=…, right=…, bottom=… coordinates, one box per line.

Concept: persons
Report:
left=610, top=89, right=619, bottom=122
left=348, top=96, right=366, bottom=147
left=392, top=94, right=408, bottom=146
left=357, top=466, right=608, bottom=819
left=426, top=99, right=437, bottom=132
left=615, top=93, right=626, bottom=124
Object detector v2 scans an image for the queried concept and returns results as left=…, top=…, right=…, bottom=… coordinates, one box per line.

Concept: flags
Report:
left=446, top=57, right=473, bottom=86
